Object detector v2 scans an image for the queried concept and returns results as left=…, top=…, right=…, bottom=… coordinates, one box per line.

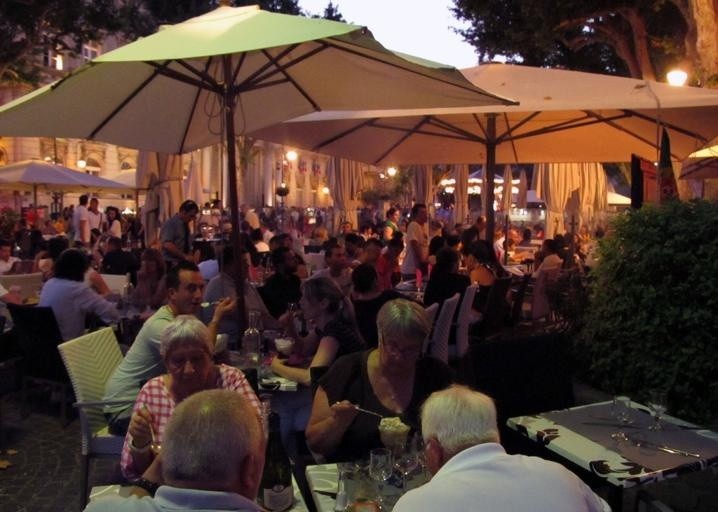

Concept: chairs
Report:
left=0, top=231, right=573, bottom=365
left=6, top=302, right=77, bottom=430
left=58, top=326, right=136, bottom=512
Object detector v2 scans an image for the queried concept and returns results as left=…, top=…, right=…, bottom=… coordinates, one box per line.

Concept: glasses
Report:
left=381, top=327, right=423, bottom=356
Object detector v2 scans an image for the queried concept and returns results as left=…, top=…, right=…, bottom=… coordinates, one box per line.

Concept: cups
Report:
left=275, top=336, right=296, bottom=370
left=287, top=302, right=298, bottom=312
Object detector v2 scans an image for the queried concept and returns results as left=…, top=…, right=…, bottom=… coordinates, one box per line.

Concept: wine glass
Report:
left=335, top=418, right=431, bottom=512
left=611, top=395, right=632, bottom=442
left=646, top=398, right=667, bottom=431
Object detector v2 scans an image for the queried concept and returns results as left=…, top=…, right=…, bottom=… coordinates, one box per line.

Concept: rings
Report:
left=138, top=403, right=147, bottom=409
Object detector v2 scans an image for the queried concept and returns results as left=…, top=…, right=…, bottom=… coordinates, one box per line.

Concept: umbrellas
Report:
left=244, top=62, right=718, bottom=249
left=102, top=167, right=148, bottom=214
left=0, top=158, right=136, bottom=230
left=679, top=145, right=718, bottom=179
left=0, top=6, right=521, bottom=351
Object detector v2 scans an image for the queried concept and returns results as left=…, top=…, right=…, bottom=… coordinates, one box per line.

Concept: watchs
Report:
left=133, top=476, right=159, bottom=498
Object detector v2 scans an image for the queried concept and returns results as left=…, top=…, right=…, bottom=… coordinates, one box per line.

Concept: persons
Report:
left=1, top=194, right=613, bottom=512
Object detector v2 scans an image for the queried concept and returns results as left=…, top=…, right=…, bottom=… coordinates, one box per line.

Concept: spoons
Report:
left=142, top=402, right=162, bottom=453
left=200, top=296, right=241, bottom=307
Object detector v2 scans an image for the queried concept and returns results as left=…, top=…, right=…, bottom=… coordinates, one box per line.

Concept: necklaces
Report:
left=379, top=364, right=414, bottom=400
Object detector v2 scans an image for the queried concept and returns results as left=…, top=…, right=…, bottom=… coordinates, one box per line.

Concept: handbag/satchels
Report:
left=90, top=229, right=101, bottom=244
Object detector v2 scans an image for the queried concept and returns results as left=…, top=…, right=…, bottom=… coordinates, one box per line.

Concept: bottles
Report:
left=123, top=273, right=134, bottom=296
left=242, top=311, right=262, bottom=382
left=256, top=410, right=294, bottom=511
left=256, top=256, right=272, bottom=286
left=8, top=281, right=22, bottom=302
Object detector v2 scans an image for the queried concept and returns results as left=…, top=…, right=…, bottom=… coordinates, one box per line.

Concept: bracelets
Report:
left=210, top=319, right=219, bottom=326
left=129, top=441, right=150, bottom=454
